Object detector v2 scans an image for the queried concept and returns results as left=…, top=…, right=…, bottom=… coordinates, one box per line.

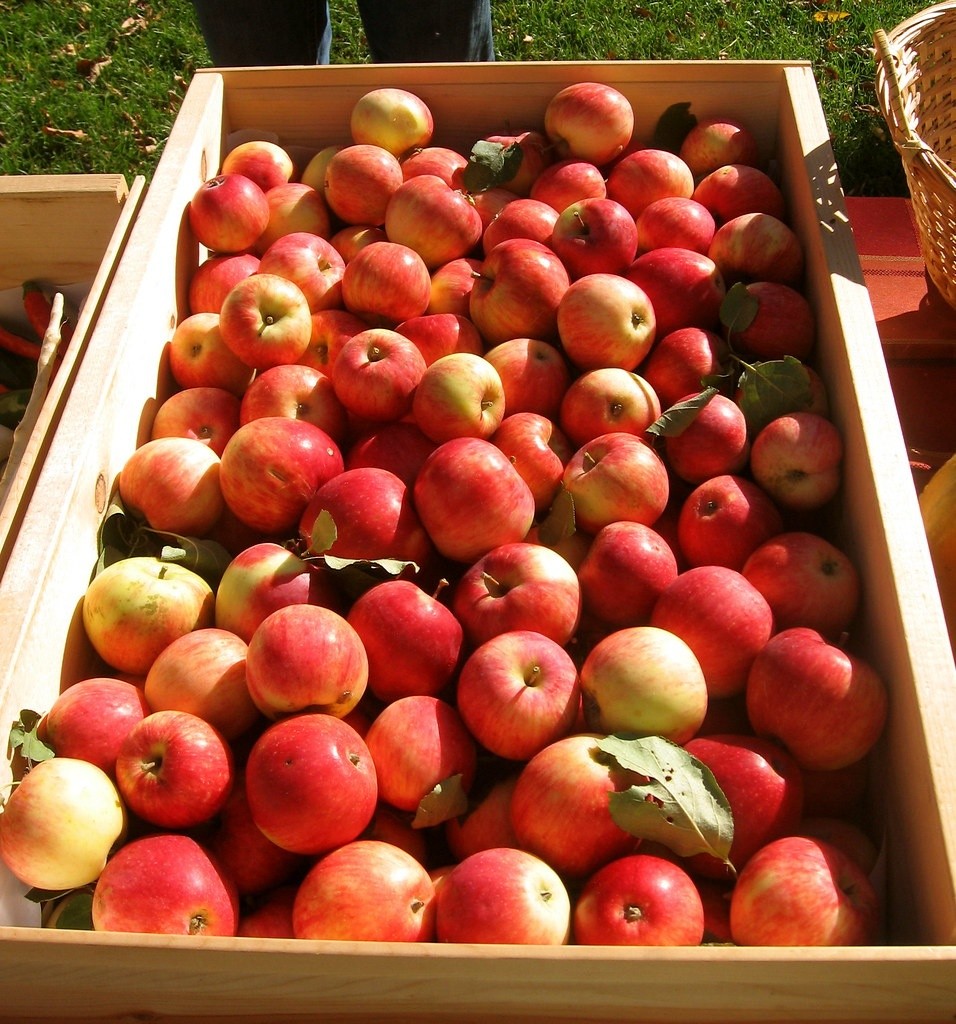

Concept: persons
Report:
left=185, top=0, right=495, bottom=68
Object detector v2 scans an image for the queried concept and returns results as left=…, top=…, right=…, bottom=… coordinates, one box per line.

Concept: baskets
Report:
left=872, top=0, right=956, bottom=312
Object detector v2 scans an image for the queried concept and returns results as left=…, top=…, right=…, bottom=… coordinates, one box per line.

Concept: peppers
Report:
left=1, top=388, right=35, bottom=426
left=0, top=322, right=61, bottom=381
left=22, top=279, right=74, bottom=358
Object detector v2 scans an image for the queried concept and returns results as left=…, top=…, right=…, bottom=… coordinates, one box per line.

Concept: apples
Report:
left=0, top=82, right=904, bottom=948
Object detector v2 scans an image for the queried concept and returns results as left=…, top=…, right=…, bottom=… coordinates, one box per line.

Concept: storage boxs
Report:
left=0, top=175, right=150, bottom=567
left=0, top=56, right=956, bottom=1024
